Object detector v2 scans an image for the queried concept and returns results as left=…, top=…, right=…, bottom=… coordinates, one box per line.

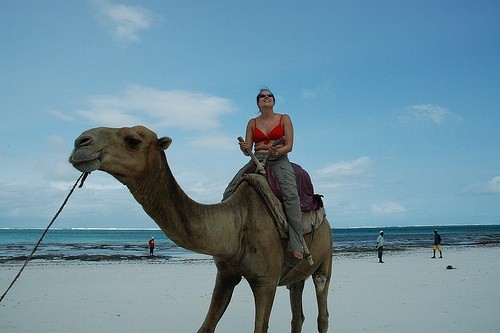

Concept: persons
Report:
left=432, top=229, right=442, bottom=258
left=224, top=89, right=304, bottom=260
left=376, top=231, right=385, bottom=263
left=149, top=237, right=154, bottom=256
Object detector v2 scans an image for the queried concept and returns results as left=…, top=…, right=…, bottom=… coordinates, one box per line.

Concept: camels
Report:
left=68, top=125, right=333, bottom=333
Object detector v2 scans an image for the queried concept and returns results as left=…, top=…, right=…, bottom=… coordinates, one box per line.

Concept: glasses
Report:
left=259, top=93, right=273, bottom=98
